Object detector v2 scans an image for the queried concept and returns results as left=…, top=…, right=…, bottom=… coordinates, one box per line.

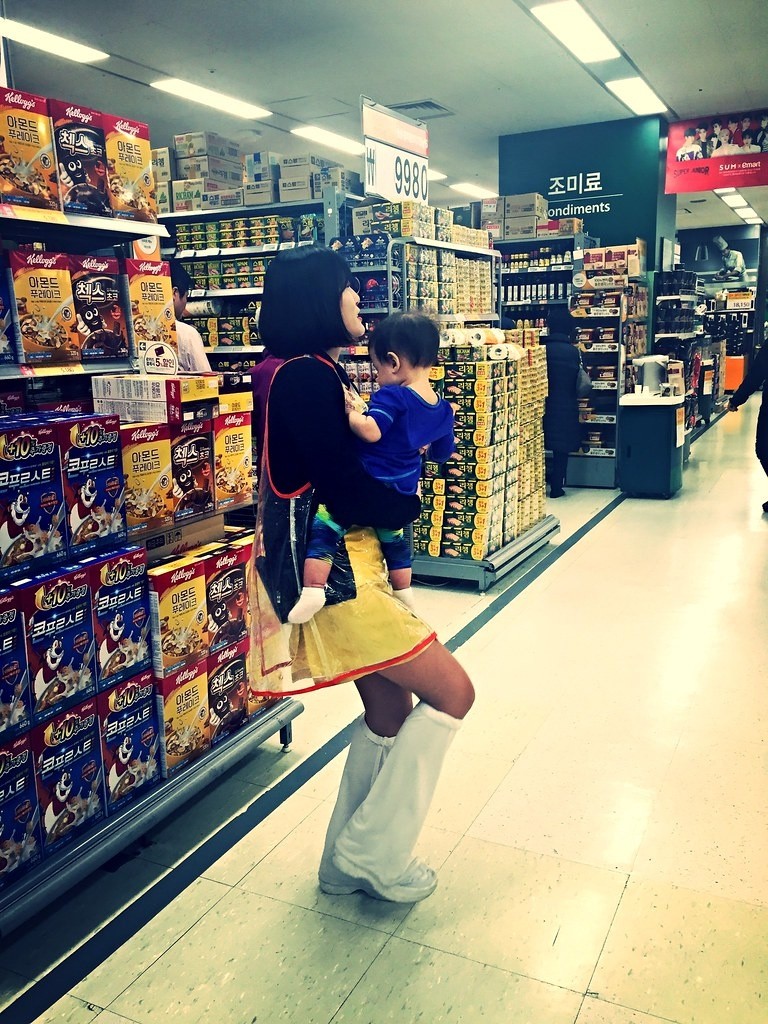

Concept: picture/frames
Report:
left=661, top=237, right=674, bottom=272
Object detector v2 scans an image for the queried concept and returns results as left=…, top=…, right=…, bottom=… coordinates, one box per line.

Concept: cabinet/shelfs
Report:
left=0, top=203, right=304, bottom=944
left=337, top=189, right=501, bottom=364
left=160, top=185, right=339, bottom=374
left=490, top=233, right=601, bottom=320
left=562, top=285, right=630, bottom=488
left=655, top=295, right=698, bottom=344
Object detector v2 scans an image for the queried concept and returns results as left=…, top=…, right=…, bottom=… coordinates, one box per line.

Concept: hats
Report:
left=712, top=235, right=728, bottom=252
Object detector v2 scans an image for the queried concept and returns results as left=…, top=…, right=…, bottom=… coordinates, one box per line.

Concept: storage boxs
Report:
left=0, top=85, right=648, bottom=894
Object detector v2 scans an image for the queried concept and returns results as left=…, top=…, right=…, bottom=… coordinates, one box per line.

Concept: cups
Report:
left=635, top=385, right=650, bottom=394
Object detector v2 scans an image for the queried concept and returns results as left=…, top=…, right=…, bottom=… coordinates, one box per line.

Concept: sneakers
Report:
left=319, top=879, right=387, bottom=902
left=331, top=846, right=438, bottom=903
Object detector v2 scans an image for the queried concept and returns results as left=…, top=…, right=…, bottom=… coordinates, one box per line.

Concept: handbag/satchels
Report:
left=576, top=348, right=594, bottom=399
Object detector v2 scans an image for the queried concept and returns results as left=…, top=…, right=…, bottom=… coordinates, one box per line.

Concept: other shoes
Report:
left=550, top=488, right=565, bottom=498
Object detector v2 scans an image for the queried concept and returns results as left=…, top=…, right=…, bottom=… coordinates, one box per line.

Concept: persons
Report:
left=542, top=309, right=586, bottom=498
left=249, top=246, right=475, bottom=904
left=676, top=114, right=768, bottom=162
left=162, top=259, right=212, bottom=371
left=728, top=339, right=768, bottom=512
left=493, top=302, right=516, bottom=329
left=287, top=313, right=454, bottom=623
left=715, top=236, right=747, bottom=281
